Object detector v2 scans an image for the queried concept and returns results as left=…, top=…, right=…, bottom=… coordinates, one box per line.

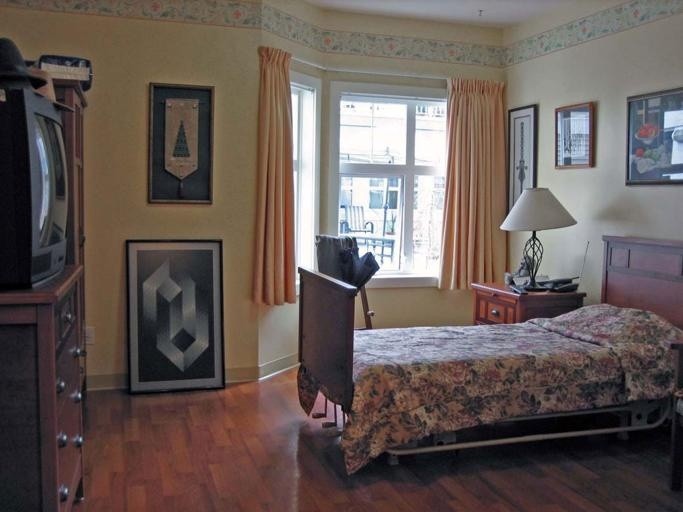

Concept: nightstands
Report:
left=471, top=281, right=586, bottom=325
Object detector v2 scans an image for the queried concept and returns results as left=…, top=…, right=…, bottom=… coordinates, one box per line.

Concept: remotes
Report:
left=509, top=284, right=528, bottom=295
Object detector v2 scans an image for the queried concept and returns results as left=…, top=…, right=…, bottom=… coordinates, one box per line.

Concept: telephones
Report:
left=543, top=276, right=580, bottom=292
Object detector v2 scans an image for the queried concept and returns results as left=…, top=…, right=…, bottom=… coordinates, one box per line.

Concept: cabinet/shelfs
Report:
left=51, top=78, right=88, bottom=391
left=0, top=264, right=85, bottom=512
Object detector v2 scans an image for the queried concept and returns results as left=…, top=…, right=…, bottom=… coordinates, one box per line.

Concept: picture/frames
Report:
left=126, top=238, right=225, bottom=394
left=554, top=102, right=593, bottom=169
left=507, top=104, right=537, bottom=214
left=148, top=82, right=215, bottom=205
left=625, top=87, right=683, bottom=185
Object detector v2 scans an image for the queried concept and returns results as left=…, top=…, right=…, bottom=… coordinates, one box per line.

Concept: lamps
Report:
left=499, top=187, right=577, bottom=292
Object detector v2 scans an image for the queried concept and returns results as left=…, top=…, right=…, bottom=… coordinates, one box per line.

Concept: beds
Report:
left=298, top=235, right=683, bottom=491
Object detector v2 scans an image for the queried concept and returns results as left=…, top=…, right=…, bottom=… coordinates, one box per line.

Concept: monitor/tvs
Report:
left=0, top=87, right=70, bottom=290
